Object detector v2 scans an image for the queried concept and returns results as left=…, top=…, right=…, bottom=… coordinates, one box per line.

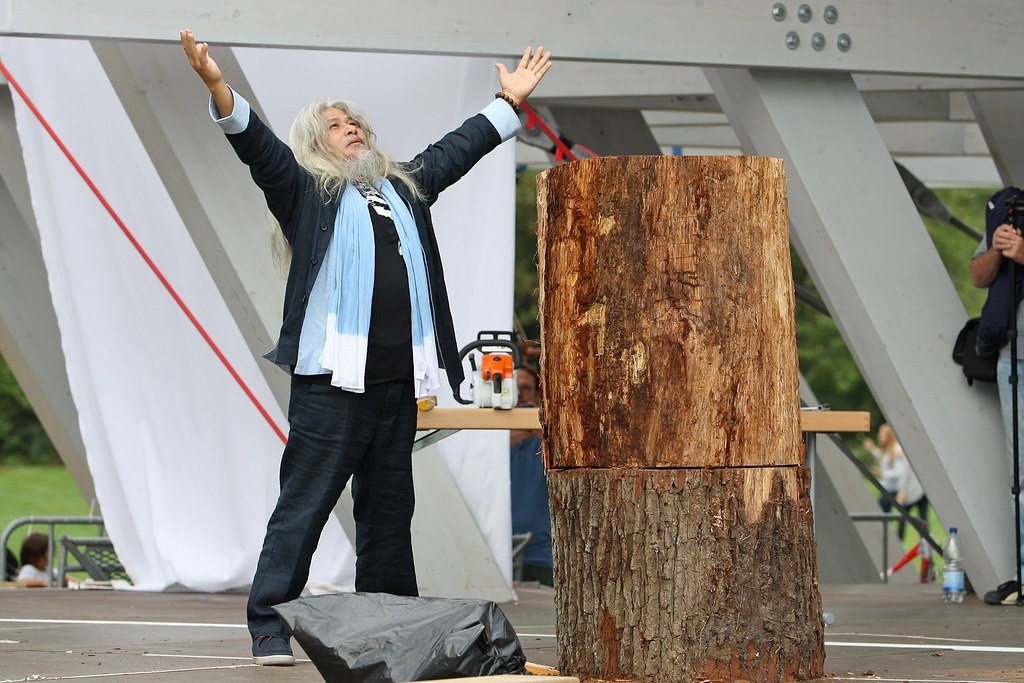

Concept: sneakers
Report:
left=984, top=580, right=1024, bottom=605
left=251, top=635, right=295, bottom=666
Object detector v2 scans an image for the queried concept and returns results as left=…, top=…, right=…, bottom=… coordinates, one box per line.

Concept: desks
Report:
left=410, top=405, right=871, bottom=548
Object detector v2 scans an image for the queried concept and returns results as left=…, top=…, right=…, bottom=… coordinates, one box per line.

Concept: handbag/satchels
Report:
left=953, top=316, right=1001, bottom=386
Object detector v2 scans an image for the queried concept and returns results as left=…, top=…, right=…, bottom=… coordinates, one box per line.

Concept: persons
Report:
left=509, top=318, right=556, bottom=587
left=968, top=223, right=1024, bottom=605
left=181, top=28, right=553, bottom=664
left=2, top=531, right=81, bottom=590
left=862, top=424, right=931, bottom=543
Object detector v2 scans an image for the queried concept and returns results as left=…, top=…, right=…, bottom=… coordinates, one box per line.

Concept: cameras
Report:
left=1003, top=197, right=1024, bottom=217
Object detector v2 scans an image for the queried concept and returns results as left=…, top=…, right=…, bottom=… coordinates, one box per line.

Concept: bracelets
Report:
left=495, top=91, right=520, bottom=112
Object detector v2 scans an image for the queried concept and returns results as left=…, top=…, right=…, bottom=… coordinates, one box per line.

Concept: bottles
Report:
left=823, top=612, right=834, bottom=626
left=942, top=527, right=965, bottom=603
